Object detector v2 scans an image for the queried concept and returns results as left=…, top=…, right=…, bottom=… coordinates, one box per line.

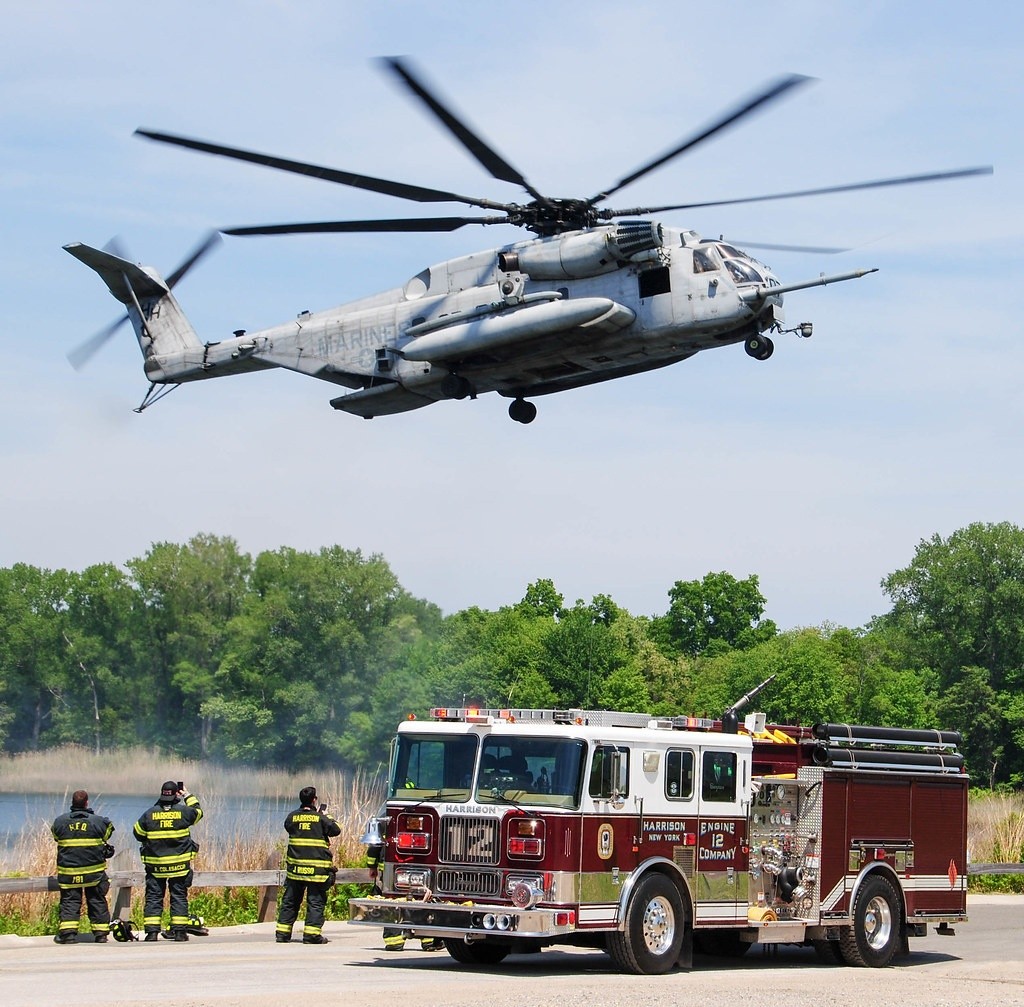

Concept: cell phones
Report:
left=177, top=782, right=183, bottom=793
left=319, top=804, right=326, bottom=814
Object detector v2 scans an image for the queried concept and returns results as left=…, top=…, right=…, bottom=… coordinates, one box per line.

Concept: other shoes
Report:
left=145, top=931, right=157, bottom=941
left=422, top=942, right=446, bottom=950
left=175, top=930, right=189, bottom=941
left=54, top=932, right=76, bottom=944
left=276, top=937, right=292, bottom=942
left=386, top=944, right=403, bottom=951
left=95, top=935, right=107, bottom=943
left=303, top=935, right=328, bottom=944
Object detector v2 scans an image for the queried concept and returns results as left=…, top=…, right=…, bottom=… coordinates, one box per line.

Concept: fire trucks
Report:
left=349, top=673, right=973, bottom=974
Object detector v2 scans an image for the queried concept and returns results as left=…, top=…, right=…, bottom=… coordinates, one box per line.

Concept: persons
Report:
left=132, top=781, right=203, bottom=941
left=366, top=812, right=445, bottom=951
left=50, top=790, right=115, bottom=944
left=275, top=786, right=341, bottom=945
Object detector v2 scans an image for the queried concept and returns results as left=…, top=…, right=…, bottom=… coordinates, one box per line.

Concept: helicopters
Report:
left=63, top=58, right=997, bottom=424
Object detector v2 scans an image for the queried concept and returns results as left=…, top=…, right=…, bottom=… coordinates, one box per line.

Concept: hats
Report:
left=160, top=781, right=177, bottom=795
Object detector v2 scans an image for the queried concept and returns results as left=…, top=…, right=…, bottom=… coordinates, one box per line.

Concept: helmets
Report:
left=188, top=915, right=200, bottom=930
left=113, top=923, right=130, bottom=942
